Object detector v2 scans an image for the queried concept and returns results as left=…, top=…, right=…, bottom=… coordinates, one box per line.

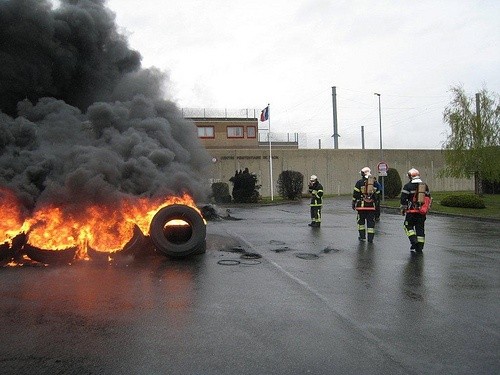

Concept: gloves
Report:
left=401, top=210, right=405, bottom=216
left=352, top=204, right=355, bottom=210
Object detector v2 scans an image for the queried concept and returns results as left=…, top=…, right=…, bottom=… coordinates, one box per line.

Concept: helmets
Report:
left=359, top=167, right=370, bottom=176
left=407, top=168, right=419, bottom=178
left=310, top=175, right=317, bottom=180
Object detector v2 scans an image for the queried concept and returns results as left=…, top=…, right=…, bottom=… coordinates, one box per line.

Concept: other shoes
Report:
left=308, top=221, right=315, bottom=226
left=359, top=237, right=365, bottom=239
left=312, top=222, right=320, bottom=228
left=415, top=249, right=422, bottom=252
left=411, top=243, right=418, bottom=249
left=368, top=240, right=372, bottom=243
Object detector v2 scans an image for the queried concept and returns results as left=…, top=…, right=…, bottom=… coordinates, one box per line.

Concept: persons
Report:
left=307, top=175, right=324, bottom=227
left=400, top=168, right=432, bottom=252
left=352, top=167, right=380, bottom=243
left=373, top=177, right=381, bottom=221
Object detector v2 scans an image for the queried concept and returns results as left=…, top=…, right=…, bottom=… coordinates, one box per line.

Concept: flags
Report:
left=260, top=106, right=270, bottom=122
